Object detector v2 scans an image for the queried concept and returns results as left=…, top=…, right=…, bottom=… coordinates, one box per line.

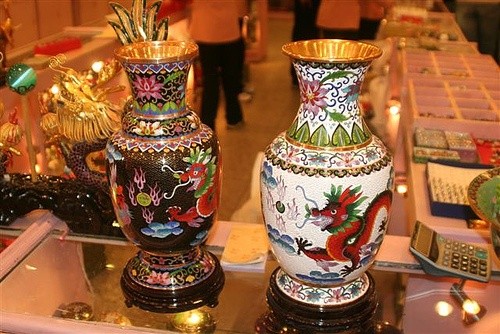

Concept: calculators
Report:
left=409, top=221, right=491, bottom=283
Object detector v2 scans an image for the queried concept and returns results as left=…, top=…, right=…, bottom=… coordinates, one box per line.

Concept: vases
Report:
left=260, top=35, right=394, bottom=333
left=104, top=40, right=225, bottom=313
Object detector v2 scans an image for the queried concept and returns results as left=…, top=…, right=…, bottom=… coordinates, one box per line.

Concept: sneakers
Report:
left=227, top=121, right=244, bottom=129
left=237, top=92, right=251, bottom=102
left=243, top=82, right=255, bottom=93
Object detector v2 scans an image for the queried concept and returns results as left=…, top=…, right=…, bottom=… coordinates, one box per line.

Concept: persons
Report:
left=315, top=0, right=361, bottom=43
left=289, top=0, right=321, bottom=91
left=188, top=0, right=248, bottom=132
left=360, top=2, right=391, bottom=39
